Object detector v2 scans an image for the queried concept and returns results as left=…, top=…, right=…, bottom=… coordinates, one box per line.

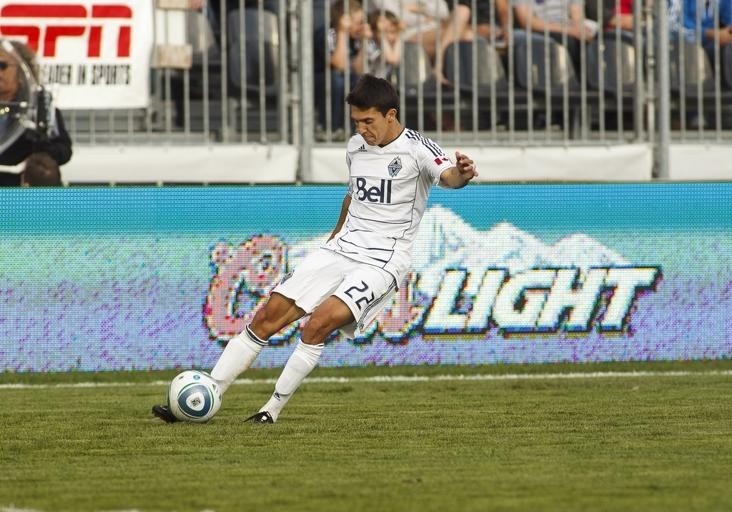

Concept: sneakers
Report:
left=151, top=404, right=178, bottom=424
left=244, top=411, right=274, bottom=425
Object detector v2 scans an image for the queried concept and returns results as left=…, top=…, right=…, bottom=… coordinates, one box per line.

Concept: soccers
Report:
left=167, top=370, right=222, bottom=424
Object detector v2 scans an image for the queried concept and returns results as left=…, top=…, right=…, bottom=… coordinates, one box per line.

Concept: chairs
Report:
left=151, top=0, right=732, bottom=137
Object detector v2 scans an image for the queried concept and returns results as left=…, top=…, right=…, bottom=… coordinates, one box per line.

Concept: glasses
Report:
left=0, top=60, right=18, bottom=70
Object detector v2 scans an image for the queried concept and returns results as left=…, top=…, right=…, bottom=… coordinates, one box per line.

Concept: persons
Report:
left=146, top=68, right=479, bottom=426
left=2, top=39, right=72, bottom=189
left=17, top=153, right=61, bottom=189
left=151, top=0, right=731, bottom=135
